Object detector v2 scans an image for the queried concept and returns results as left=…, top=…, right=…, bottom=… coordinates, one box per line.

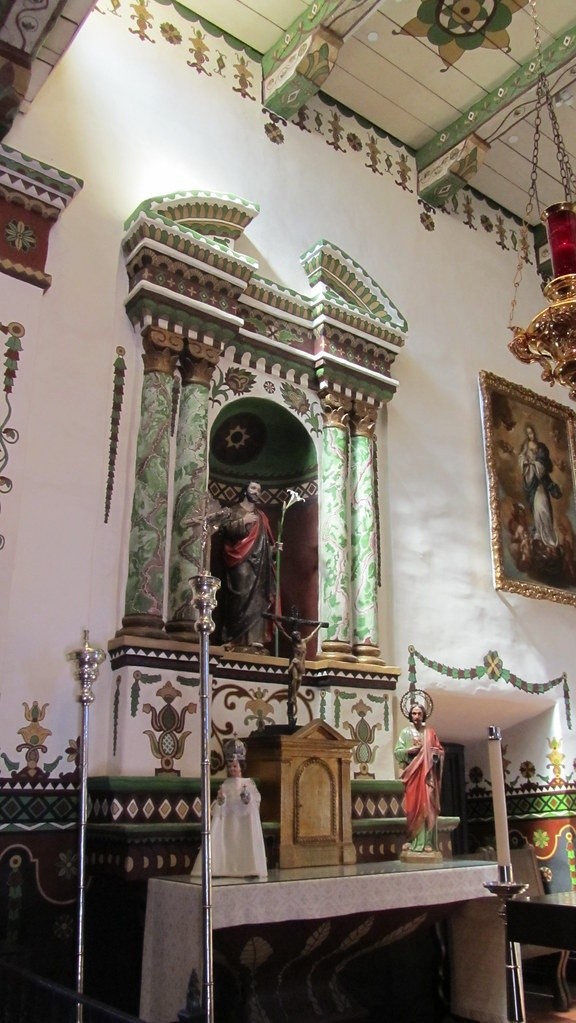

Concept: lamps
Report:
left=503, top=0, right=576, bottom=394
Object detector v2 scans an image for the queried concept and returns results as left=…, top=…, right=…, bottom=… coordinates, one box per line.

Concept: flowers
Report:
left=276, top=486, right=305, bottom=656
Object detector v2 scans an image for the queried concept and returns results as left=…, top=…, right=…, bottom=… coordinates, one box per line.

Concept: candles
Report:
left=487, top=720, right=514, bottom=883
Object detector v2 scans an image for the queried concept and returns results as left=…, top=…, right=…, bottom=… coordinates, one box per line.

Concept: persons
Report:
left=219, top=480, right=284, bottom=648
left=189, top=732, right=268, bottom=875
left=394, top=704, right=444, bottom=851
left=270, top=614, right=322, bottom=704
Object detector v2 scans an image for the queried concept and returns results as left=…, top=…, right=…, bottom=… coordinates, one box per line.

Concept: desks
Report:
left=137, top=851, right=526, bottom=1023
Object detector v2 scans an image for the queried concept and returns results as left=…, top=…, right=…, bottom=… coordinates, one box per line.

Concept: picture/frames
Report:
left=474, top=371, right=576, bottom=605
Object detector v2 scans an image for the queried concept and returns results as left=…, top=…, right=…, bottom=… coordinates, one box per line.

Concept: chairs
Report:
left=460, top=847, right=573, bottom=1013
